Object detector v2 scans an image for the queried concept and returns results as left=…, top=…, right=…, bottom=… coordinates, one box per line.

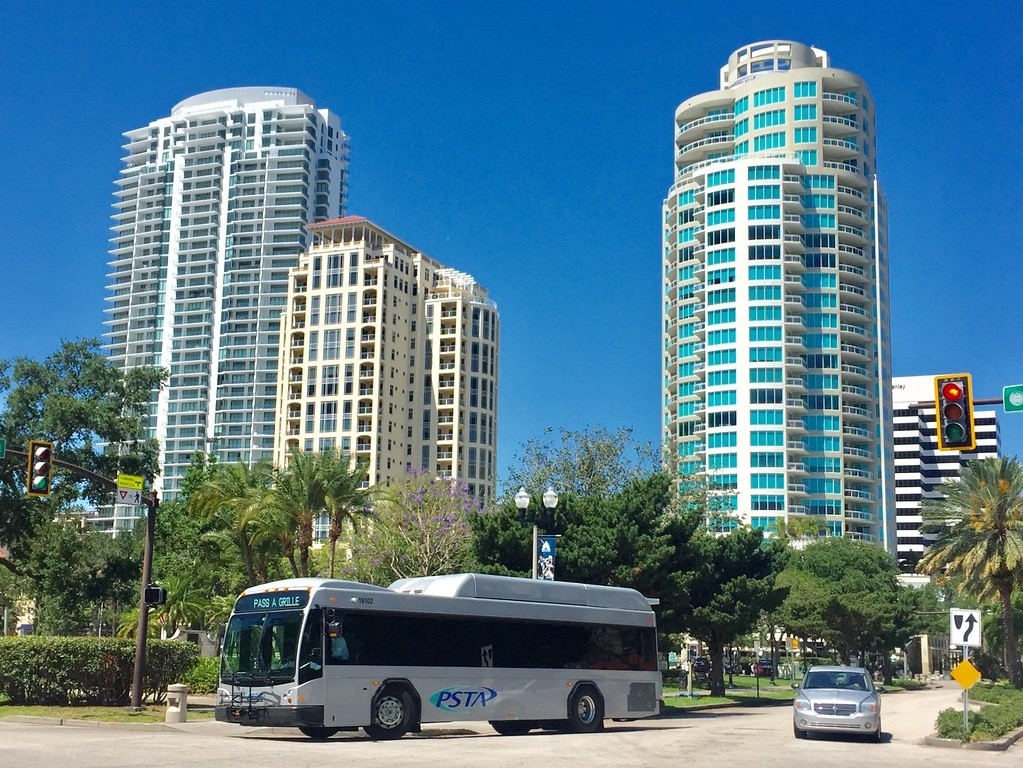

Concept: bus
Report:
left=216, top=573, right=663, bottom=740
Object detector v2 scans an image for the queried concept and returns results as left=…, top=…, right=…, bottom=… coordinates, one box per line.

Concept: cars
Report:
left=791, top=664, right=884, bottom=742
left=725, top=662, right=744, bottom=674
left=691, top=658, right=710, bottom=672
left=753, top=658, right=779, bottom=677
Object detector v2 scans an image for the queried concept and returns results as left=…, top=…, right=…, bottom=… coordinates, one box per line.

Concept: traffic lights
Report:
left=942, top=383, right=964, bottom=441
left=33, top=447, right=51, bottom=489
left=145, top=588, right=160, bottom=602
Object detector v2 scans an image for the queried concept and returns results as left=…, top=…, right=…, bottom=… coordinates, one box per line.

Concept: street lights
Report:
left=514, top=486, right=558, bottom=578
